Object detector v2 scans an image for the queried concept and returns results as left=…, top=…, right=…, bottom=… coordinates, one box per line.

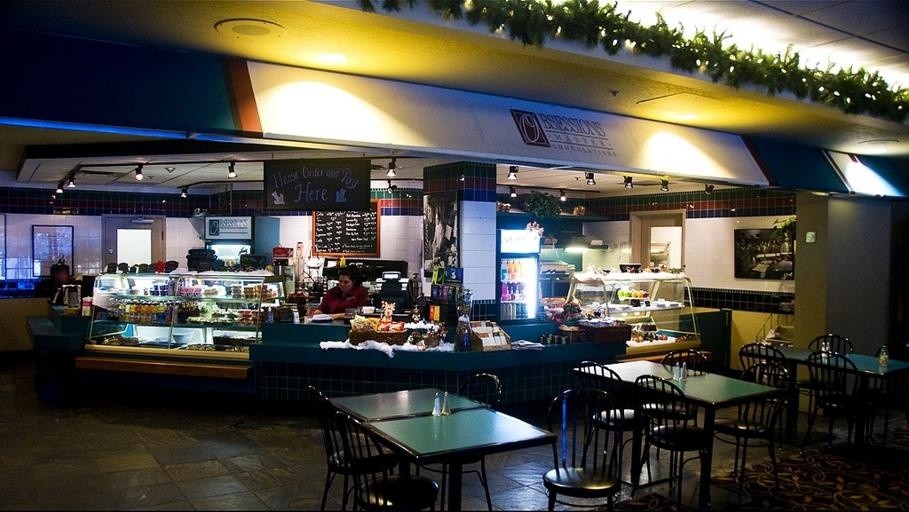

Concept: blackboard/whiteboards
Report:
left=264, top=159, right=370, bottom=210
left=311, top=198, right=381, bottom=258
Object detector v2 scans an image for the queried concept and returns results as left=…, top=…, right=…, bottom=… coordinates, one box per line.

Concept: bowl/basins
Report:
left=619, top=263, right=642, bottom=273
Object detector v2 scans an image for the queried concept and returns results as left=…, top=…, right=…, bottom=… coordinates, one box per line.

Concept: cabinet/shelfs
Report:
left=564, top=272, right=702, bottom=370
left=73, top=272, right=286, bottom=413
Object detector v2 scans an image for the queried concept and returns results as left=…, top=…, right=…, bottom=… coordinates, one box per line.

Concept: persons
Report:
left=314, top=266, right=369, bottom=314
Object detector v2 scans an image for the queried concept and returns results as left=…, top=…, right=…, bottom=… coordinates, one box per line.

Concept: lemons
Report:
left=631, top=291, right=643, bottom=298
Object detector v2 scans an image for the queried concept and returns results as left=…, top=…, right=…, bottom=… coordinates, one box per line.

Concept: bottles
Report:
left=266, top=304, right=274, bottom=322
left=75, top=264, right=83, bottom=282
left=431, top=392, right=442, bottom=417
left=879, top=345, right=890, bottom=367
left=500, top=259, right=528, bottom=320
left=255, top=331, right=265, bottom=344
left=81, top=296, right=94, bottom=318
left=106, top=297, right=178, bottom=324
left=821, top=341, right=831, bottom=358
left=673, top=361, right=681, bottom=381
left=442, top=391, right=452, bottom=416
left=144, top=278, right=175, bottom=296
left=679, top=361, right=688, bottom=381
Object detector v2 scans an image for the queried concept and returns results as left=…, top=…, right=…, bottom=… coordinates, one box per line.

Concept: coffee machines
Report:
left=271, top=247, right=296, bottom=296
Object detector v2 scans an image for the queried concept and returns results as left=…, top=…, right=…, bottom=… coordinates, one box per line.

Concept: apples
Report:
left=618, top=290, right=631, bottom=297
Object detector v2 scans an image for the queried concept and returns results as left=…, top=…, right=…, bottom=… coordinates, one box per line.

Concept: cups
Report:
left=539, top=334, right=569, bottom=345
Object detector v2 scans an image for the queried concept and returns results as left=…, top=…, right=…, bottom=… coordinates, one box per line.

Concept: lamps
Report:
left=659, top=178, right=669, bottom=192
left=386, top=158, right=397, bottom=177
left=704, top=183, right=714, bottom=195
left=623, top=176, right=634, bottom=189
left=180, top=186, right=189, bottom=198
left=134, top=165, right=143, bottom=181
left=506, top=166, right=519, bottom=180
left=585, top=172, right=595, bottom=186
left=387, top=179, right=397, bottom=194
left=227, top=161, right=237, bottom=178
left=56, top=174, right=76, bottom=194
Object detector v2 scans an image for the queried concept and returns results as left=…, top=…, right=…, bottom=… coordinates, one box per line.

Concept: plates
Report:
left=619, top=297, right=649, bottom=300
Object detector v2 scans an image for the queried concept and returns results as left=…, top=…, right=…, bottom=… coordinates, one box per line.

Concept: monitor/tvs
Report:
left=327, top=279, right=341, bottom=291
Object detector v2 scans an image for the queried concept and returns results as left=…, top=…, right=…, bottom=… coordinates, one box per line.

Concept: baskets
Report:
left=349, top=328, right=408, bottom=346
left=575, top=320, right=632, bottom=345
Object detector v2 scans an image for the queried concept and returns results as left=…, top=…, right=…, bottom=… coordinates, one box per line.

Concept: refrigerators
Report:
left=497, top=228, right=542, bottom=325
left=203, top=214, right=281, bottom=266
